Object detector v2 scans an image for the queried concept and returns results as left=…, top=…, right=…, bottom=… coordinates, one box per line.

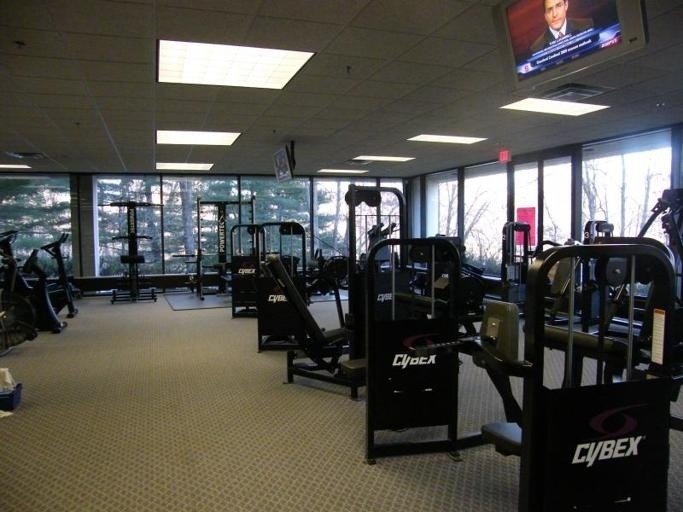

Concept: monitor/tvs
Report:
left=271, top=144, right=294, bottom=184
left=493, top=0, right=651, bottom=95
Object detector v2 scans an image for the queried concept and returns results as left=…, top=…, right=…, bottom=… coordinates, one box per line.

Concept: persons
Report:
left=527, top=0, right=594, bottom=54
